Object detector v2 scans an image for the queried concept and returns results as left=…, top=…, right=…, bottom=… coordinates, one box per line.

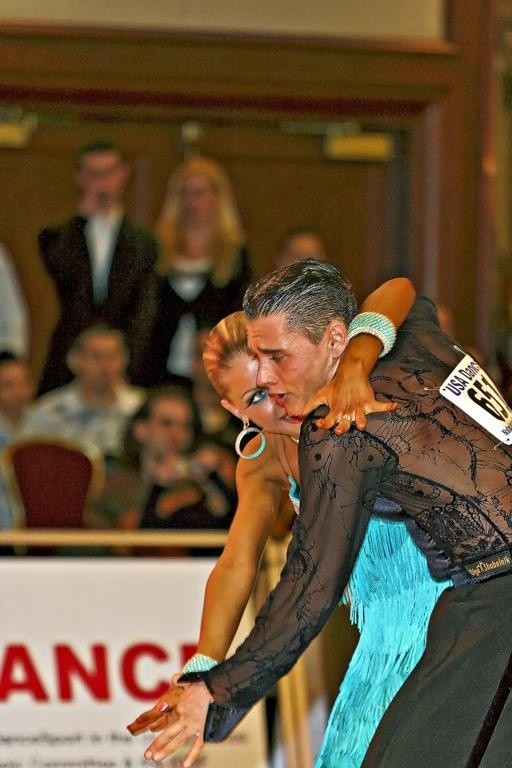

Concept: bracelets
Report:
left=345, top=311, right=396, bottom=359
left=182, top=653, right=219, bottom=678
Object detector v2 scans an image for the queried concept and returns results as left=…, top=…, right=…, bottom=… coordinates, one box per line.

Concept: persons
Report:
left=144, top=256, right=512, bottom=768
left=126, top=278, right=454, bottom=768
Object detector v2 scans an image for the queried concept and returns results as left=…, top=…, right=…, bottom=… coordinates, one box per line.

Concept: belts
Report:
left=463, top=549, right=512, bottom=585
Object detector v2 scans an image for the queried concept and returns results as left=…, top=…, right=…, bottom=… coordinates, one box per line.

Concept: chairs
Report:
left=0, top=429, right=111, bottom=558
